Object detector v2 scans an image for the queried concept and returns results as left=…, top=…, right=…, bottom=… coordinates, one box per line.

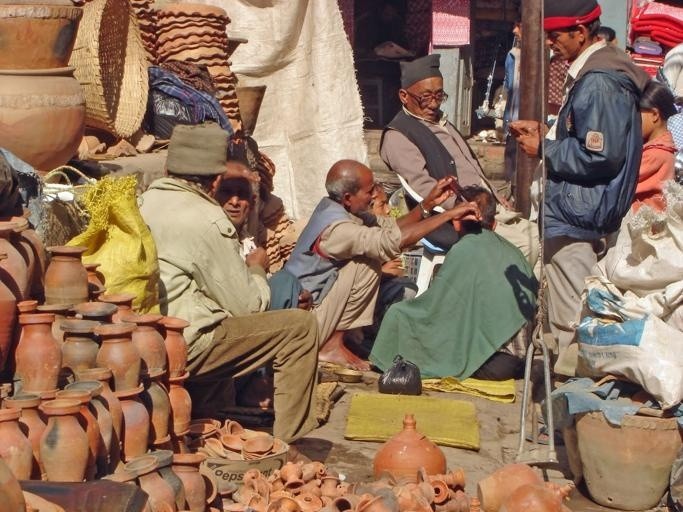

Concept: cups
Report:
left=191, top=417, right=282, bottom=460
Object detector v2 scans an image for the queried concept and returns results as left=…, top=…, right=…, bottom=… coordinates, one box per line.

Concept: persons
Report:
left=360, top=1, right=519, bottom=123
left=135, top=122, right=319, bottom=463
left=507, top=0, right=651, bottom=446
left=287, top=160, right=537, bottom=372
left=207, top=161, right=315, bottom=315
left=503, top=0, right=682, bottom=386
left=382, top=53, right=541, bottom=270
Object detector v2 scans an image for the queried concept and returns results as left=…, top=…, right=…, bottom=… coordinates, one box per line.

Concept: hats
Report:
left=541, top=0, right=602, bottom=31
left=398, top=52, right=443, bottom=89
left=165, top=120, right=229, bottom=177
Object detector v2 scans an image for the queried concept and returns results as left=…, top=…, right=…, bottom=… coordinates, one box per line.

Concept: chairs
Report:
left=396, top=175, right=445, bottom=299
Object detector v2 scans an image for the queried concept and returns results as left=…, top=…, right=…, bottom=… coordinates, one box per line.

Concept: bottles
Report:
left=497, top=95, right=504, bottom=118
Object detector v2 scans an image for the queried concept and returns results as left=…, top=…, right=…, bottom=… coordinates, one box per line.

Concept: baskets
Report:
left=78, top=0, right=152, bottom=141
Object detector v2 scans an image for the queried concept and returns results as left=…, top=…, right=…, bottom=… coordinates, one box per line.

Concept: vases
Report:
left=577, top=413, right=682, bottom=510
left=0, top=4, right=86, bottom=183
left=0, top=215, right=207, bottom=512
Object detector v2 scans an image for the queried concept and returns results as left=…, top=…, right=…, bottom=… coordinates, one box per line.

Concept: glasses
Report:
left=406, top=91, right=449, bottom=104
